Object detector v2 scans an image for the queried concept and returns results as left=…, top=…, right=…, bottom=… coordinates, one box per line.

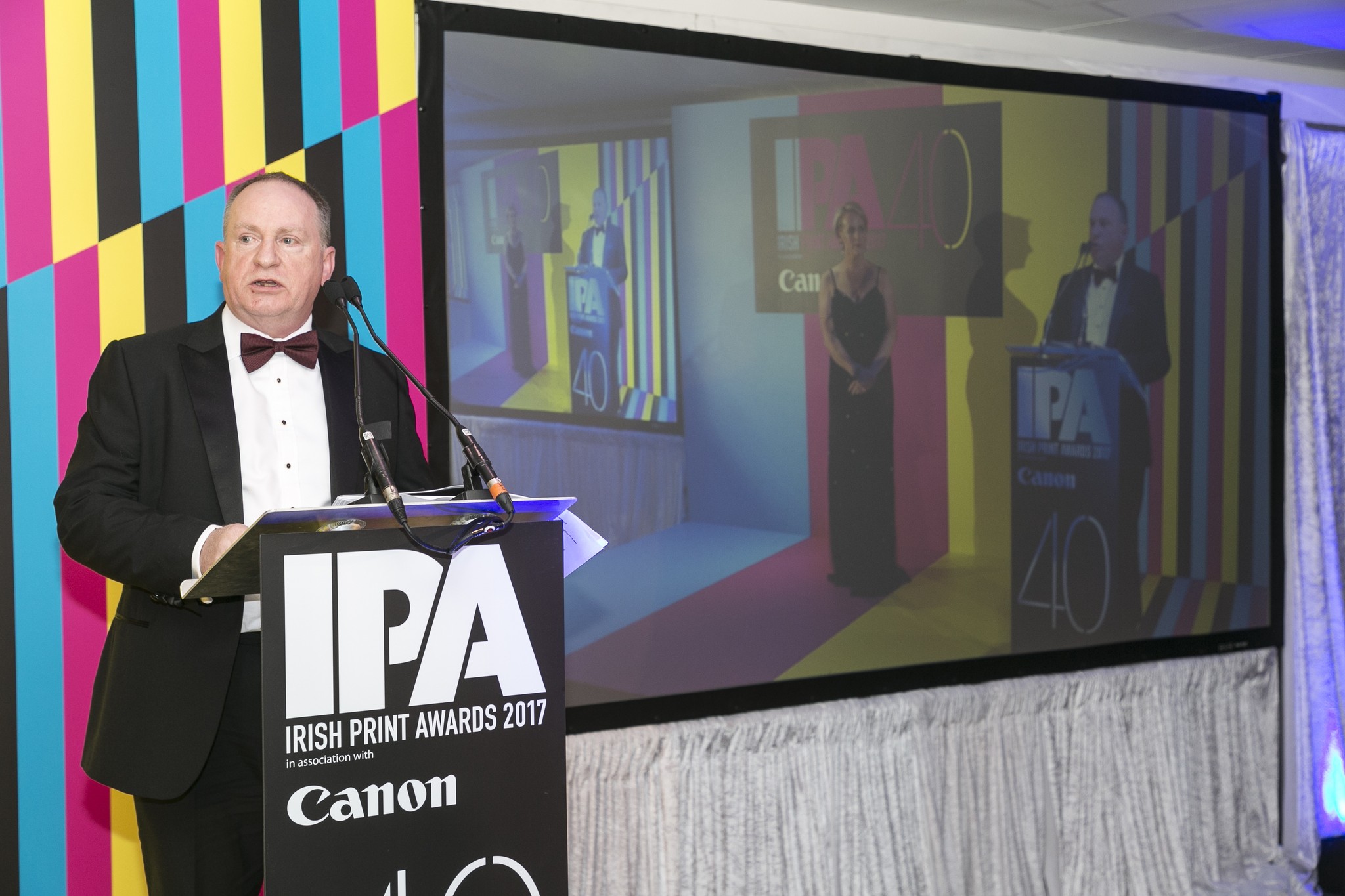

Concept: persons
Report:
left=505, top=207, right=531, bottom=363
left=51, top=174, right=439, bottom=896
left=1040, top=189, right=1172, bottom=638
left=817, top=201, right=898, bottom=596
left=577, top=187, right=627, bottom=287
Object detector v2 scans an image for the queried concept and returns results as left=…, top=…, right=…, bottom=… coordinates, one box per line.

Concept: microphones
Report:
left=1038, top=241, right=1094, bottom=351
left=324, top=276, right=515, bottom=524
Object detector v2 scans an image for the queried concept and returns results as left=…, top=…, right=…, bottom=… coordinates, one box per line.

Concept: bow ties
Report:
left=1093, top=268, right=1116, bottom=288
left=595, top=227, right=604, bottom=235
left=240, top=329, right=319, bottom=373
